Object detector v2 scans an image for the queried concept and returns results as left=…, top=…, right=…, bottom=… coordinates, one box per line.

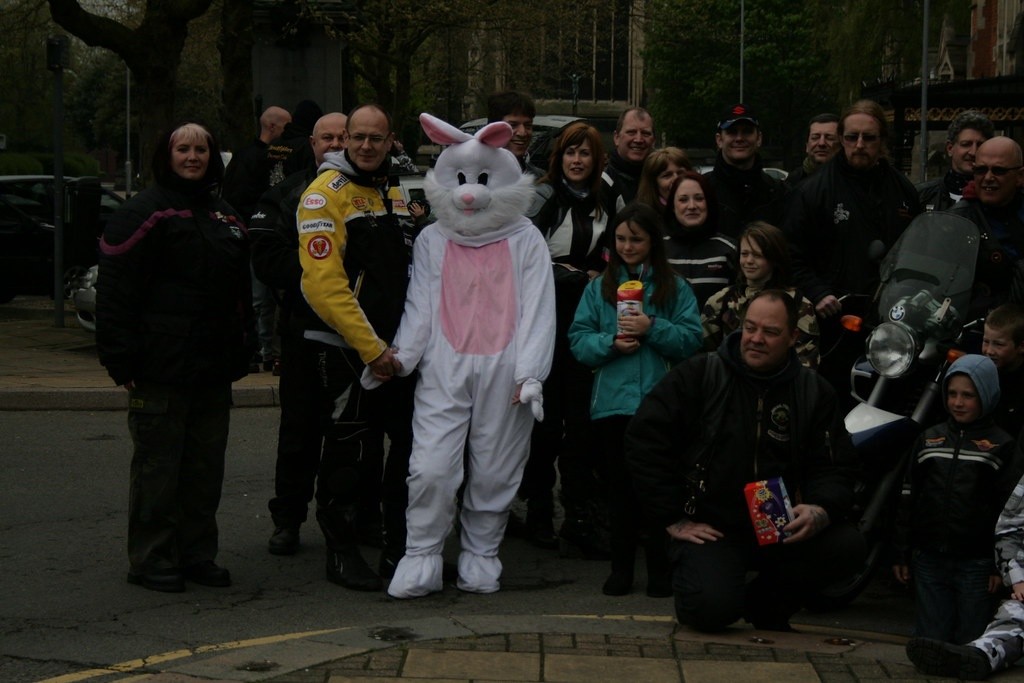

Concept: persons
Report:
left=484, top=92, right=1024, bottom=682
left=228, top=99, right=437, bottom=589
left=94, top=123, right=254, bottom=593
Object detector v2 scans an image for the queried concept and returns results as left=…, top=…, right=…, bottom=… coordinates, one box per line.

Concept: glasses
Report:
left=971, top=164, right=1022, bottom=176
left=350, top=132, right=392, bottom=145
left=842, top=134, right=882, bottom=142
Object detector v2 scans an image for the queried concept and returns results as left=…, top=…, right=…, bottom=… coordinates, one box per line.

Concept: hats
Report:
left=715, top=101, right=759, bottom=130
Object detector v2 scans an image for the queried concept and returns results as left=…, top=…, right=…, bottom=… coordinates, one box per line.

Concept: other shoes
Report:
left=268, top=528, right=300, bottom=553
left=906, top=635, right=991, bottom=680
left=603, top=529, right=676, bottom=597
left=129, top=569, right=186, bottom=592
left=326, top=553, right=383, bottom=591
left=170, top=559, right=231, bottom=587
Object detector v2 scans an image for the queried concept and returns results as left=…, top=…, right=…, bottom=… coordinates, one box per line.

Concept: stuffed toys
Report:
left=359, top=114, right=557, bottom=596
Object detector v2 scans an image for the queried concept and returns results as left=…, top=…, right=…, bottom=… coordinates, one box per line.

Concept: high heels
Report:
left=558, top=497, right=612, bottom=561
left=525, top=487, right=558, bottom=549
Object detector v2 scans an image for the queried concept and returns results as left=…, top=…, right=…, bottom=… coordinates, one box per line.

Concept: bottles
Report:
left=616, top=281, right=644, bottom=339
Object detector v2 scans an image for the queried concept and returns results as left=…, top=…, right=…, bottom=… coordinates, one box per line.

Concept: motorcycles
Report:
left=817, top=209, right=1019, bottom=614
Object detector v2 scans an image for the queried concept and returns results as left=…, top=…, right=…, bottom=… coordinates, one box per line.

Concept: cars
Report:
left=458, top=115, right=589, bottom=149
left=74, top=263, right=99, bottom=333
left=0, top=173, right=126, bottom=305
